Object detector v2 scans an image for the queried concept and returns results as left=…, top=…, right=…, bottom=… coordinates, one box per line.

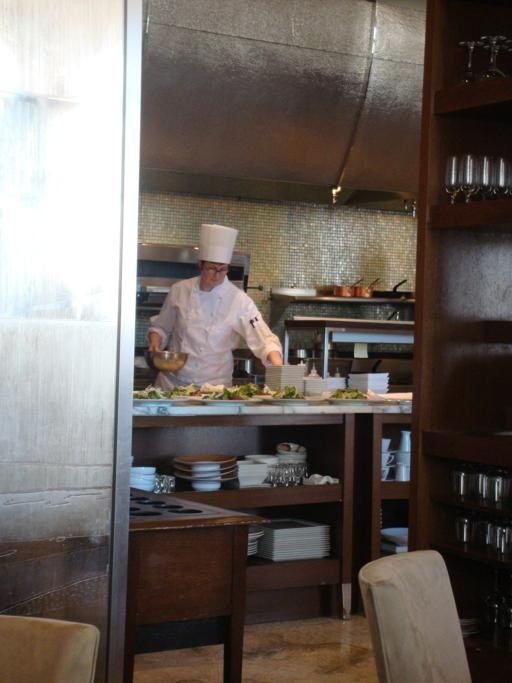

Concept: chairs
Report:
left=0, top=608, right=101, bottom=681
left=359, top=547, right=478, bottom=683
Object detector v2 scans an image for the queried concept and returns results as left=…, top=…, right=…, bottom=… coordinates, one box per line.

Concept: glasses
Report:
left=206, top=266, right=229, bottom=274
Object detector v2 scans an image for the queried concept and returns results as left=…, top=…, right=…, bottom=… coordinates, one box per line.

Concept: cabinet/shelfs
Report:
left=409, top=0, right=512, bottom=683
left=372, top=405, right=418, bottom=555
left=121, top=394, right=352, bottom=625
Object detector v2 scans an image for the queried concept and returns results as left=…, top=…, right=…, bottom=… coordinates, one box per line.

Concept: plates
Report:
left=265, top=364, right=347, bottom=394
left=262, top=398, right=325, bottom=406
left=133, top=398, right=188, bottom=407
left=348, top=372, right=390, bottom=395
left=247, top=518, right=334, bottom=563
left=174, top=454, right=238, bottom=490
left=189, top=396, right=261, bottom=405
left=328, top=397, right=371, bottom=406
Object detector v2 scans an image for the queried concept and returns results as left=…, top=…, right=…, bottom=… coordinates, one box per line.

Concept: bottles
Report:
left=395, top=432, right=411, bottom=482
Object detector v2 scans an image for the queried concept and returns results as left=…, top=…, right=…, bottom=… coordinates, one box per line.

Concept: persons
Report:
left=146, top=224, right=283, bottom=390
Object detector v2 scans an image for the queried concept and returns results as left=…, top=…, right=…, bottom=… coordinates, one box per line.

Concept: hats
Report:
left=199, top=224, right=238, bottom=264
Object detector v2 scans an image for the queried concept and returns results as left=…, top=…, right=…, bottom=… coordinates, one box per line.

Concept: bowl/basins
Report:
left=129, top=466, right=157, bottom=493
left=380, top=527, right=407, bottom=546
left=246, top=443, right=307, bottom=463
left=461, top=616, right=479, bottom=638
left=143, top=348, right=188, bottom=372
left=236, top=461, right=269, bottom=487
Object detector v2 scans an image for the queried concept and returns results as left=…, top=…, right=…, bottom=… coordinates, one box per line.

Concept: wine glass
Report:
left=458, top=33, right=512, bottom=84
left=443, top=153, right=511, bottom=202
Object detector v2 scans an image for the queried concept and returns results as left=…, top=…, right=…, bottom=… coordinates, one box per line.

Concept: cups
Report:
left=381, top=437, right=397, bottom=481
left=450, top=464, right=511, bottom=508
left=268, top=463, right=307, bottom=487
left=456, top=515, right=512, bottom=556
left=154, top=475, right=176, bottom=495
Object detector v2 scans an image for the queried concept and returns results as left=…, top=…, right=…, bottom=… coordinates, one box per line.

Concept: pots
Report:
left=289, top=349, right=318, bottom=364
left=354, top=277, right=380, bottom=297
left=333, top=278, right=364, bottom=297
left=374, top=280, right=413, bottom=298
left=234, top=358, right=256, bottom=378
